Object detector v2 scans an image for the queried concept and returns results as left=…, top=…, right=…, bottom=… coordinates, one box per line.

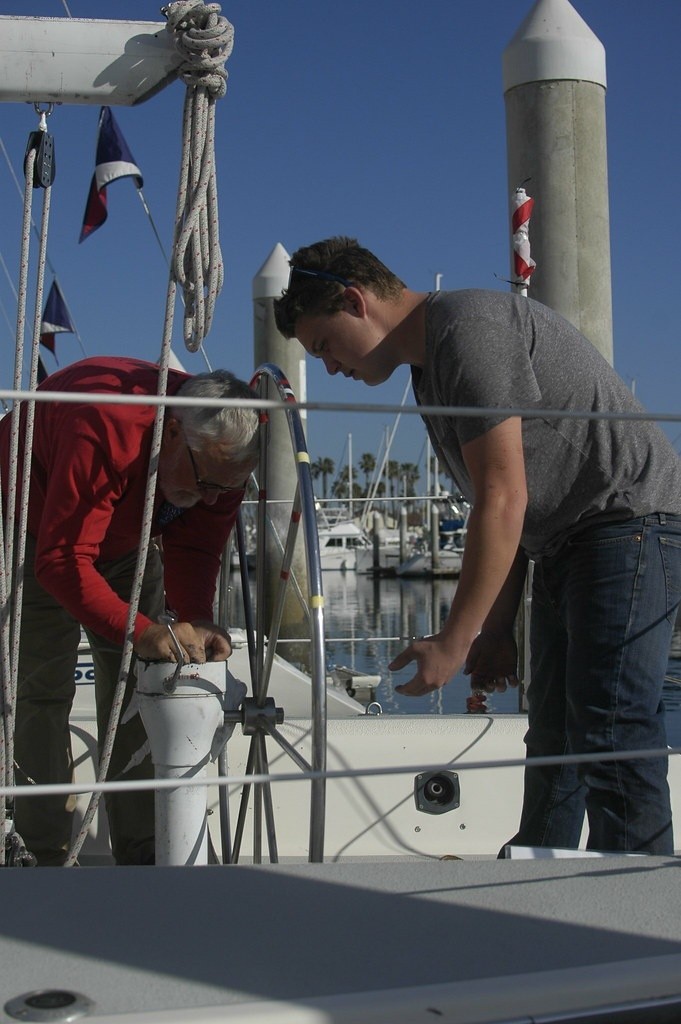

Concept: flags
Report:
left=78, top=106, right=144, bottom=243
left=39, top=281, right=75, bottom=367
left=37, top=357, right=47, bottom=385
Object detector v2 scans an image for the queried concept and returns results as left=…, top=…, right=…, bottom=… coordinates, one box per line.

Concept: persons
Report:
left=0, top=357, right=261, bottom=868
left=275, top=235, right=681, bottom=858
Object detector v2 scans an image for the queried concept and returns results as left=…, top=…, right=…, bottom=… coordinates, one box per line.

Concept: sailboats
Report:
left=0, top=0, right=679, bottom=1021
left=221, top=273, right=469, bottom=578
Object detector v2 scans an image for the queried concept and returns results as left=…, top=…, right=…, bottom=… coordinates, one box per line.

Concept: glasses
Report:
left=274, top=258, right=352, bottom=307
left=174, top=419, right=253, bottom=493
